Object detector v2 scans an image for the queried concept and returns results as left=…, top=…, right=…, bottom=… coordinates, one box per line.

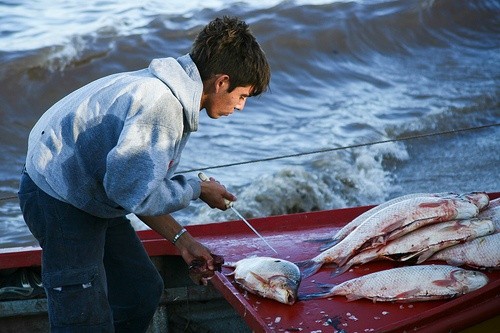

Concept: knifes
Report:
left=198, top=172, right=278, bottom=256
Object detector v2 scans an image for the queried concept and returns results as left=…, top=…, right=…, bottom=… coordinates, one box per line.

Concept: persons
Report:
left=17, top=16, right=273, bottom=333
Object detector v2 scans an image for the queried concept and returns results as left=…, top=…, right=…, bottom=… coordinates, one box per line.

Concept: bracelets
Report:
left=170, top=227, right=187, bottom=245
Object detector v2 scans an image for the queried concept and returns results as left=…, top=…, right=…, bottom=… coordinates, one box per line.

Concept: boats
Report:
left=0, top=191, right=499, bottom=329
